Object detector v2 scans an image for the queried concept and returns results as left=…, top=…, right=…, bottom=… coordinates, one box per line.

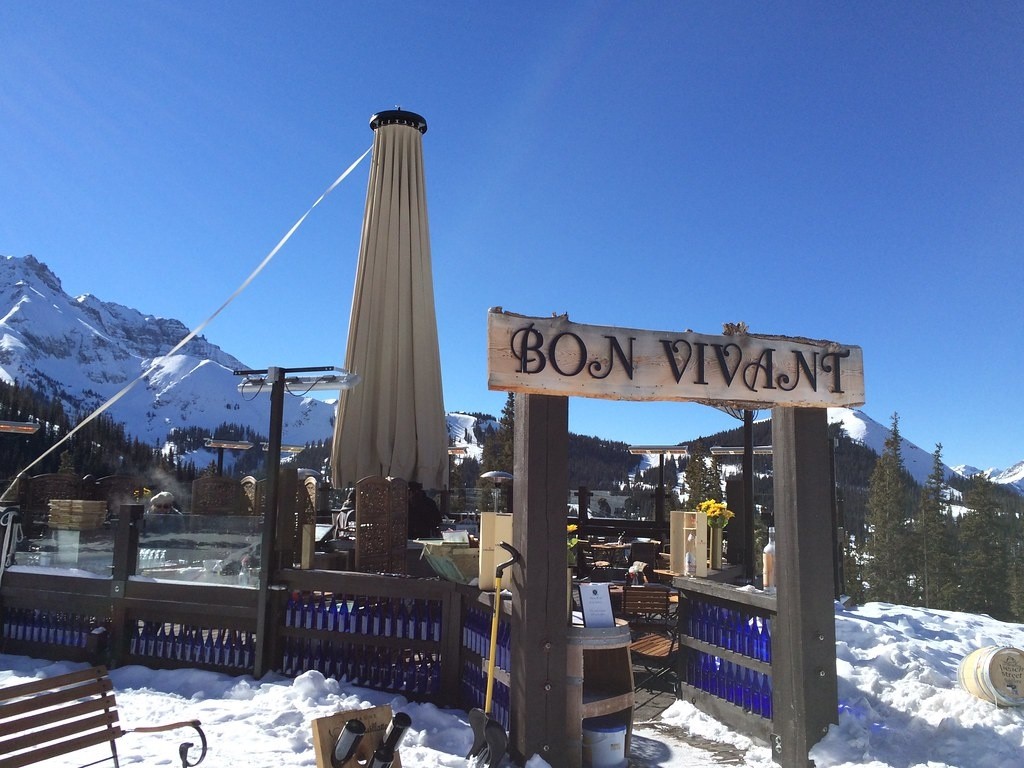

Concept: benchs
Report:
left=0, top=666, right=207, bottom=768
left=569, top=561, right=682, bottom=696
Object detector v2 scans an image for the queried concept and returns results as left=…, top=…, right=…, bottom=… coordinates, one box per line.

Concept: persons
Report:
left=408, top=478, right=444, bottom=539
left=333, top=485, right=357, bottom=541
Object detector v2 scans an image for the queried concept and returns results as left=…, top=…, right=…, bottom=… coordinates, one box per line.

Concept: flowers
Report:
left=567, top=523, right=579, bottom=564
left=697, top=498, right=735, bottom=530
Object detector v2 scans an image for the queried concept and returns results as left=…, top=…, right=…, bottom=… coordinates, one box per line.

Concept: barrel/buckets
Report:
left=957, top=645, right=1024, bottom=708
left=582, top=719, right=626, bottom=768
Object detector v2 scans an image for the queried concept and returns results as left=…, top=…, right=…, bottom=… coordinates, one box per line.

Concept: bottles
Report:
left=378, top=712, right=412, bottom=754
left=331, top=718, right=366, bottom=768
left=366, top=747, right=395, bottom=767
left=684, top=533, right=697, bottom=577
left=762, top=527, right=776, bottom=595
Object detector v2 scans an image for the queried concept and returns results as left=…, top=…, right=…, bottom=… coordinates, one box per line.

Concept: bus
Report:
left=449, top=512, right=481, bottom=540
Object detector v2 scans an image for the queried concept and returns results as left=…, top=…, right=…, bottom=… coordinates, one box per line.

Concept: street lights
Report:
left=481, top=470, right=515, bottom=511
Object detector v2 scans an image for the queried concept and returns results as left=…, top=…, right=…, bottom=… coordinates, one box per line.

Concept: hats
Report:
left=407, top=481, right=423, bottom=491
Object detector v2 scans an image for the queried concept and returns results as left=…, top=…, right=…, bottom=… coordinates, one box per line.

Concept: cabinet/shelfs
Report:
left=669, top=510, right=708, bottom=579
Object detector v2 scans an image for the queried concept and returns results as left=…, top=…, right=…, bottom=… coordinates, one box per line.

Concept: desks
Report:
left=609, top=585, right=623, bottom=594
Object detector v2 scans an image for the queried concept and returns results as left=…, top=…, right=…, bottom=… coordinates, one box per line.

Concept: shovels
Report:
left=465, top=541, right=521, bottom=768
